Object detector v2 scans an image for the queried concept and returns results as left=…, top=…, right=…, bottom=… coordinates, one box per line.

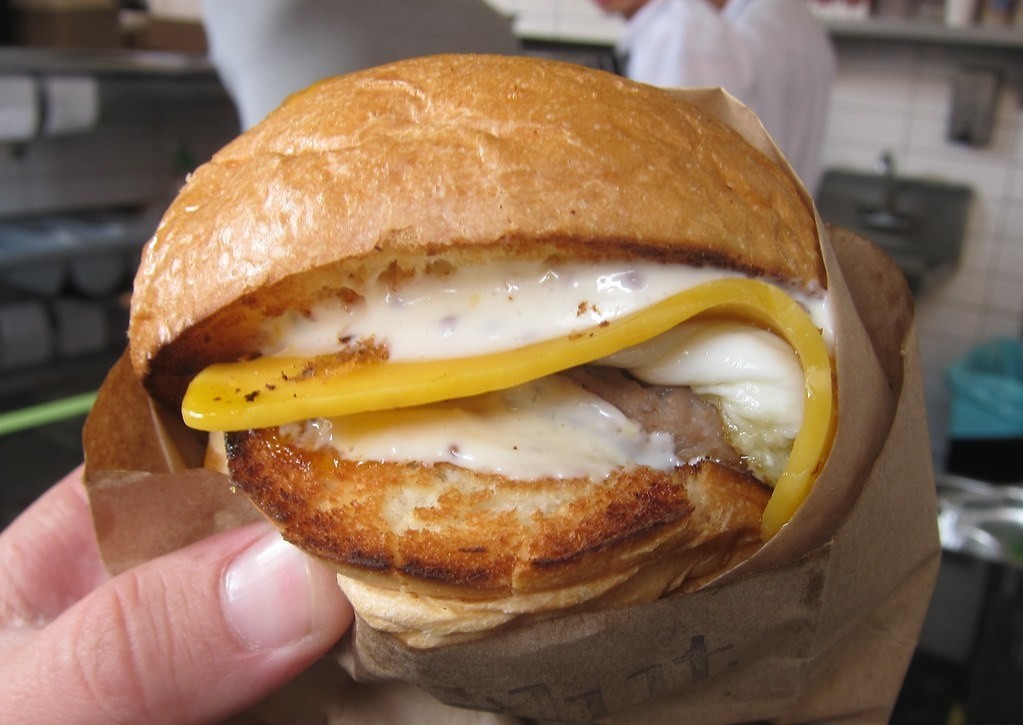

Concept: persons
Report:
left=1, top=453, right=358, bottom=724
left=592, top=0, right=836, bottom=210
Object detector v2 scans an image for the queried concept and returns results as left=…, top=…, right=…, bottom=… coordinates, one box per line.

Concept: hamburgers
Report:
left=126, top=51, right=834, bottom=651
